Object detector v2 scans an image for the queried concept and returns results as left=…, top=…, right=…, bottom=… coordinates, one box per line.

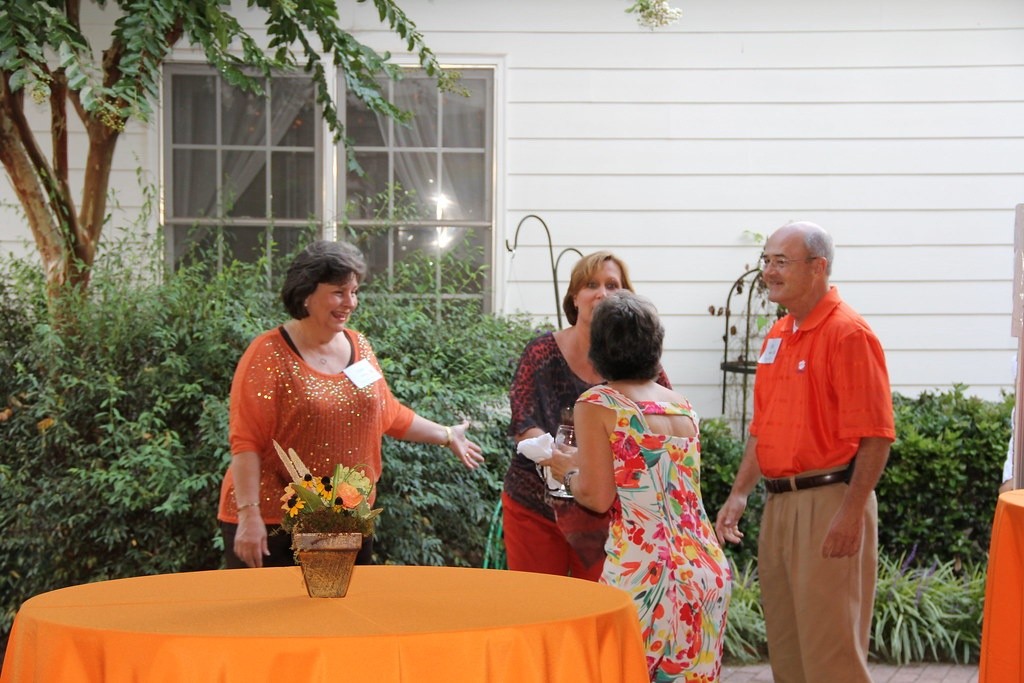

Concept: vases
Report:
left=292, top=532, right=362, bottom=599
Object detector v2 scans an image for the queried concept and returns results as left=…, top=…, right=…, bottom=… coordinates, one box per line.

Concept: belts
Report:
left=763, top=469, right=853, bottom=493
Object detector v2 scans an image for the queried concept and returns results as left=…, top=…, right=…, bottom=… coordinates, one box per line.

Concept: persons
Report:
left=537, top=289, right=733, bottom=683
left=714, top=218, right=895, bottom=683
left=501, top=250, right=674, bottom=584
left=214, top=239, right=485, bottom=570
left=998, top=405, right=1016, bottom=496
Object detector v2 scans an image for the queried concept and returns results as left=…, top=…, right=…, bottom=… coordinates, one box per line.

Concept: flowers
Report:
left=267, top=439, right=384, bottom=560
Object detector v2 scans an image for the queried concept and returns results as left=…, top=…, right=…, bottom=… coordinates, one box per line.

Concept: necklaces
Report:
left=291, top=322, right=341, bottom=364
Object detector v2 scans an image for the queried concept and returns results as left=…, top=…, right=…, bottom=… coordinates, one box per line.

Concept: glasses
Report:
left=758, top=256, right=817, bottom=270
left=535, top=462, right=564, bottom=492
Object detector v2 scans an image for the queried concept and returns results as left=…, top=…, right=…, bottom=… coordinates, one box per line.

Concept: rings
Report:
left=465, top=452, right=470, bottom=457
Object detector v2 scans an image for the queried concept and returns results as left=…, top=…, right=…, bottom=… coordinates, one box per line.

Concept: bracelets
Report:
left=439, top=426, right=452, bottom=448
left=563, top=468, right=579, bottom=495
left=237, top=501, right=261, bottom=515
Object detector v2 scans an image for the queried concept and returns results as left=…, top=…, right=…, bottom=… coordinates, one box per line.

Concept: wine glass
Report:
left=548, top=425, right=578, bottom=498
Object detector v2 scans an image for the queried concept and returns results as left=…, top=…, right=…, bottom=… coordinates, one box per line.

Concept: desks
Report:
left=0, top=565, right=650, bottom=683
left=978, top=489, right=1024, bottom=683
